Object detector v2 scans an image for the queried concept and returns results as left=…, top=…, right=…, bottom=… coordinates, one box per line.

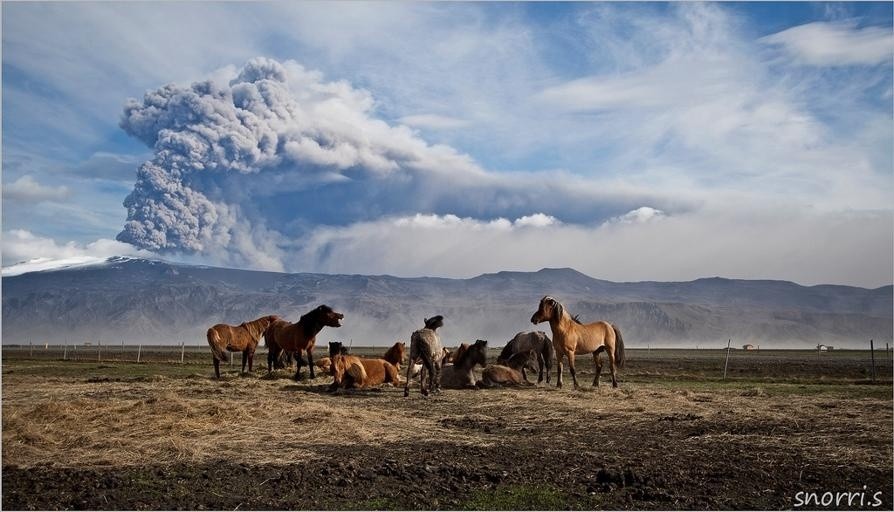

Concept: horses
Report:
left=315, top=342, right=417, bottom=392
left=404, top=316, right=553, bottom=397
left=208, top=305, right=344, bottom=382
left=531, top=296, right=625, bottom=390
